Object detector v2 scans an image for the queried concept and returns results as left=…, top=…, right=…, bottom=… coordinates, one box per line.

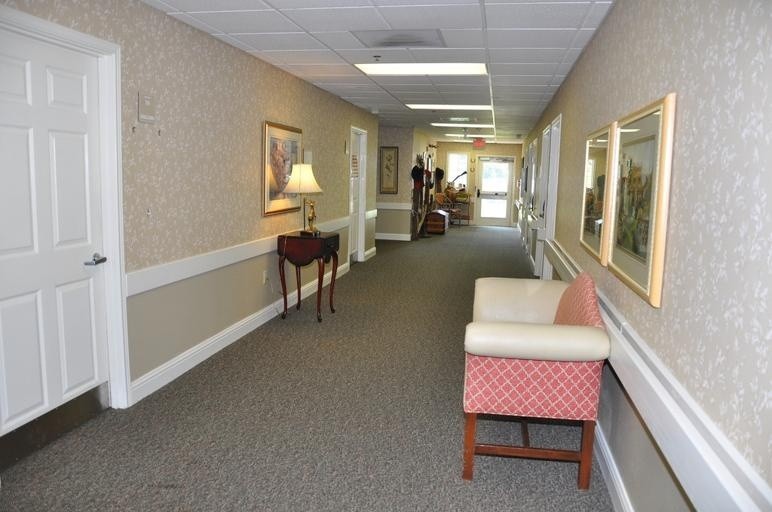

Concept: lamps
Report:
left=281, top=163, right=323, bottom=236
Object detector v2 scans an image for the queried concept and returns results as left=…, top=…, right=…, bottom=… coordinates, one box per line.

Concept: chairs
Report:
left=462, top=271, right=610, bottom=489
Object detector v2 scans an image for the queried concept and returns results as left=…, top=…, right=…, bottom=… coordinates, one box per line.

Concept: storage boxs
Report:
left=425, top=209, right=450, bottom=235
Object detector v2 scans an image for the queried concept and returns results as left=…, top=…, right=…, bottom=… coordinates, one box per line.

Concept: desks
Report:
left=277, top=231, right=340, bottom=322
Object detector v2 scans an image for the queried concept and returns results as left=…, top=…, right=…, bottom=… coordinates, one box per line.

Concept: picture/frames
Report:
left=380, top=146, right=399, bottom=195
left=577, top=91, right=677, bottom=310
left=261, top=120, right=303, bottom=216
